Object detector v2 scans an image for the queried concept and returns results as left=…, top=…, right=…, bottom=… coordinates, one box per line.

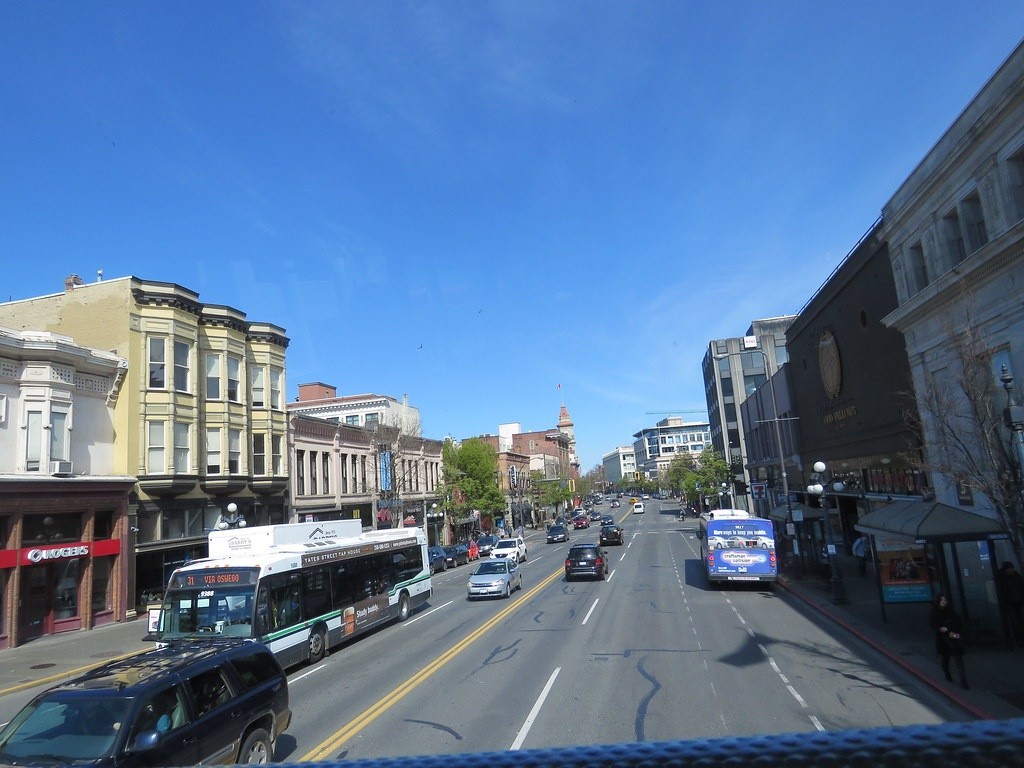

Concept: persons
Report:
left=508, top=526, right=512, bottom=538
left=998, top=561, right=1024, bottom=647
left=928, top=592, right=969, bottom=690
left=852, top=537, right=869, bottom=580
left=500, top=527, right=504, bottom=538
left=888, top=559, right=920, bottom=580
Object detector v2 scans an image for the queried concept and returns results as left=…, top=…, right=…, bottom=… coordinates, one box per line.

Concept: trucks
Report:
left=166, top=519, right=362, bottom=632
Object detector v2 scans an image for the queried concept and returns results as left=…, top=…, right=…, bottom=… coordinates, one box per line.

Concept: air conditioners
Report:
left=50, top=461, right=73, bottom=474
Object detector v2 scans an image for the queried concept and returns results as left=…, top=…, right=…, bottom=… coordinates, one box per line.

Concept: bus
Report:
left=156, top=525, right=434, bottom=671
left=696, top=509, right=778, bottom=590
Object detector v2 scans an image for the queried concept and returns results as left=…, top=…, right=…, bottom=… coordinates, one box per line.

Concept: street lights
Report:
left=518, top=457, right=544, bottom=538
left=426, top=503, right=443, bottom=546
left=713, top=349, right=802, bottom=581
left=537, top=462, right=555, bottom=496
left=673, top=467, right=703, bottom=512
left=806, top=461, right=849, bottom=605
left=718, top=483, right=732, bottom=509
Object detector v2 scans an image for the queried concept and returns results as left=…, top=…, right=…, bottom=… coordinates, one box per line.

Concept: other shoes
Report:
left=961, top=681, right=969, bottom=690
left=946, top=673, right=953, bottom=682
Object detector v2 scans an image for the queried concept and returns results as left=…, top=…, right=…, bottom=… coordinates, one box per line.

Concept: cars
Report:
left=427, top=546, right=447, bottom=576
left=546, top=525, right=569, bottom=544
left=466, top=557, right=522, bottom=601
left=590, top=512, right=603, bottom=521
left=573, top=516, right=590, bottom=529
left=443, top=545, right=470, bottom=569
left=475, top=534, right=500, bottom=555
left=459, top=540, right=481, bottom=562
left=555, top=492, right=667, bottom=526
left=600, top=514, right=614, bottom=525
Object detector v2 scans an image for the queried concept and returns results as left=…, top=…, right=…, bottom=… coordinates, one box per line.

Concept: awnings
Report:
left=524, top=503, right=533, bottom=509
left=518, top=503, right=527, bottom=510
left=512, top=504, right=520, bottom=512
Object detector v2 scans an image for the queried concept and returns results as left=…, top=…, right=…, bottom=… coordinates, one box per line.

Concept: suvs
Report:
left=600, top=525, right=624, bottom=546
left=0, top=635, right=293, bottom=768
left=565, top=543, right=609, bottom=582
left=490, top=537, right=528, bottom=564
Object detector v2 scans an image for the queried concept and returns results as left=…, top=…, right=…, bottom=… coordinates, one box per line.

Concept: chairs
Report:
left=168, top=688, right=185, bottom=730
